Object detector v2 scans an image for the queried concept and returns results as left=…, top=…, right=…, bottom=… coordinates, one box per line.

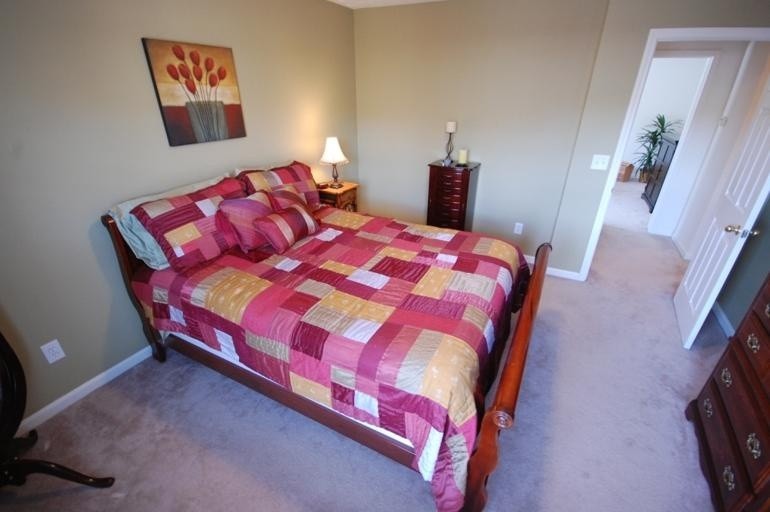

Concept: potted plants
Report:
left=632, top=112, right=682, bottom=182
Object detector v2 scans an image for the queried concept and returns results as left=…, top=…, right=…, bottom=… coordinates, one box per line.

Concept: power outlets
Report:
left=40, top=339, right=66, bottom=364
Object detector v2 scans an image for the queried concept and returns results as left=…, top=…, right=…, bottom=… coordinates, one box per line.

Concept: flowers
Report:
left=165, top=44, right=227, bottom=140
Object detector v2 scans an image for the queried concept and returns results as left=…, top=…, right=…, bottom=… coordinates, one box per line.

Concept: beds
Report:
left=101, top=164, right=551, bottom=512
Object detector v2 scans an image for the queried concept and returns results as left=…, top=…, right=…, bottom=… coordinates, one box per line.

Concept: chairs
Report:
left=0, top=331, right=116, bottom=488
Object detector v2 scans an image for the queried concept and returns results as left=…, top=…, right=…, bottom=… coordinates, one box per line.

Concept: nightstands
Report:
left=316, top=181, right=359, bottom=213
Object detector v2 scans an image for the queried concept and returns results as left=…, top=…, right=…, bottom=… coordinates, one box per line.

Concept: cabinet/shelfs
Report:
left=426, top=160, right=481, bottom=233
left=641, top=134, right=679, bottom=214
left=684, top=272, right=770, bottom=512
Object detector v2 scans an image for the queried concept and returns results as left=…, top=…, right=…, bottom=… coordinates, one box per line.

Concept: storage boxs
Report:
left=615, top=161, right=634, bottom=181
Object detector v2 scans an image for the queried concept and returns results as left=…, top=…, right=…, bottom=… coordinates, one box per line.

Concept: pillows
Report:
left=105, top=160, right=320, bottom=276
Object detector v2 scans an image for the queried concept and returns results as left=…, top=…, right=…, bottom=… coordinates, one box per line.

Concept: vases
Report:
left=185, top=101, right=228, bottom=142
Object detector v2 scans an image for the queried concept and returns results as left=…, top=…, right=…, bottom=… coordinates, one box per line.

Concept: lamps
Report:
left=443, top=121, right=457, bottom=163
left=319, top=136, right=350, bottom=188
